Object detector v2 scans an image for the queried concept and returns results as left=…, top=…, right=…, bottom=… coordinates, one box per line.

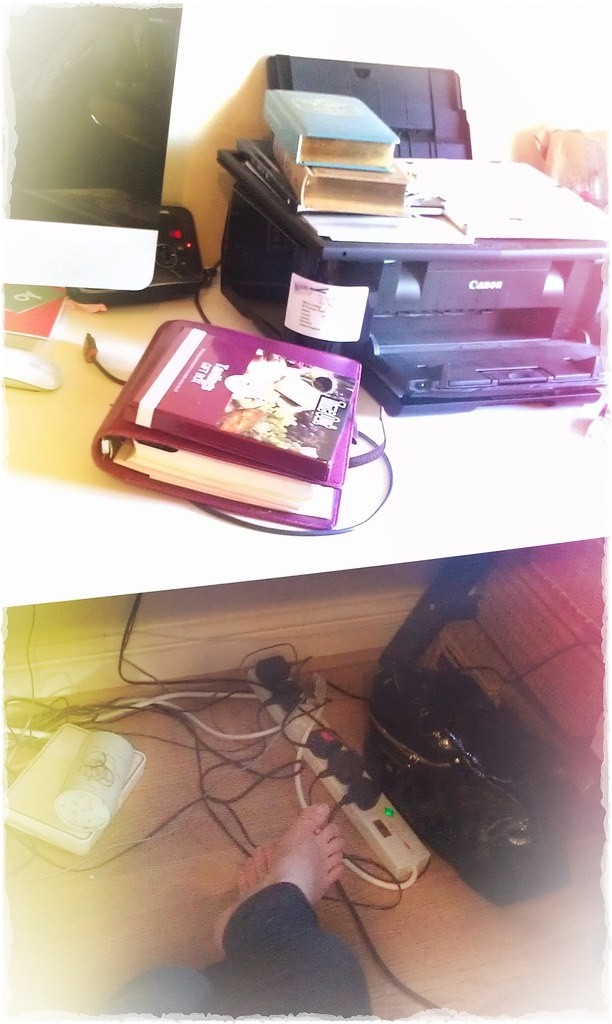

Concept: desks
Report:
left=0, top=212, right=609, bottom=740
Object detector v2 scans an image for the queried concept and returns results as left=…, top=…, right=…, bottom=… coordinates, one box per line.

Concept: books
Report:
left=90, top=316, right=361, bottom=530
left=237, top=87, right=446, bottom=220
left=3, top=283, right=69, bottom=340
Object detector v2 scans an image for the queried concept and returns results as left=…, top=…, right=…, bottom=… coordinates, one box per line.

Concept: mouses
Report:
left=0, top=346, right=62, bottom=392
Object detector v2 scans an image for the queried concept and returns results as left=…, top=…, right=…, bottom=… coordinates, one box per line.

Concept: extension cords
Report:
left=246, top=667, right=431, bottom=882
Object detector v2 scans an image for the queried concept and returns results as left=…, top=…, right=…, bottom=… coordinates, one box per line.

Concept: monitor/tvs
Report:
left=0, top=0, right=183, bottom=291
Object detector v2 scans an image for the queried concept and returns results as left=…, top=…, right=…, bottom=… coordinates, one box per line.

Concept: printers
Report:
left=214, top=54, right=608, bottom=417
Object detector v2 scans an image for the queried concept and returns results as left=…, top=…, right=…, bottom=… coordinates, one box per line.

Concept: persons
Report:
left=104, top=800, right=377, bottom=1015
